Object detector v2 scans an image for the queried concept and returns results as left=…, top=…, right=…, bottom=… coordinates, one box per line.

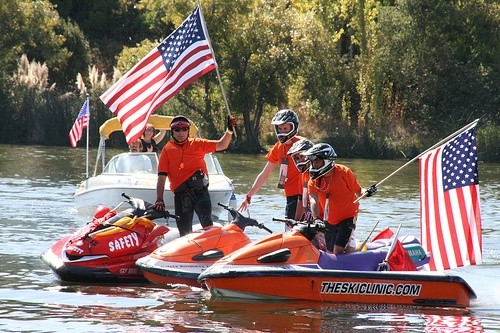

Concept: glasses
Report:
left=172, top=127, right=188, bottom=132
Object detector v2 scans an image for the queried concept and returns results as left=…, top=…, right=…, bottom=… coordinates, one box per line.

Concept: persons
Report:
left=154, top=115, right=237, bottom=237
left=130, top=139, right=142, bottom=152
left=305, top=143, right=377, bottom=255
left=287, top=138, right=326, bottom=250
left=141, top=124, right=166, bottom=153
left=238, top=109, right=307, bottom=232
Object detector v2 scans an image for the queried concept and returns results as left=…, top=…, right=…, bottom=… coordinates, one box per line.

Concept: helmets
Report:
left=286, top=139, right=314, bottom=174
left=169, top=116, right=191, bottom=131
left=300, top=143, right=337, bottom=181
left=271, top=109, right=300, bottom=144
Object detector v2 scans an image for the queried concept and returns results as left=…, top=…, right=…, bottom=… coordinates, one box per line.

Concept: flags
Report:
left=420, top=127, right=482, bottom=270
left=99, top=6, right=215, bottom=144
left=388, top=241, right=418, bottom=270
left=69, top=101, right=88, bottom=147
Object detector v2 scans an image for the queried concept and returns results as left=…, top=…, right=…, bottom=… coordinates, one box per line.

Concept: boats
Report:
left=74, top=114, right=234, bottom=224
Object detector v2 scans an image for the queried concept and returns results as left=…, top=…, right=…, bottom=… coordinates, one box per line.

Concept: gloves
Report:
left=365, top=184, right=378, bottom=197
left=153, top=200, right=165, bottom=212
left=227, top=115, right=238, bottom=132
left=313, top=218, right=326, bottom=229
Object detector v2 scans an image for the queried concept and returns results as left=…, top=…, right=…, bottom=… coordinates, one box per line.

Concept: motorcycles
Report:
left=41, top=191, right=222, bottom=286
left=198, top=217, right=479, bottom=308
left=132, top=201, right=432, bottom=300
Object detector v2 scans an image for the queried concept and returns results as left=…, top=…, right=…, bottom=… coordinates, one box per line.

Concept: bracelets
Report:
left=246, top=195, right=251, bottom=204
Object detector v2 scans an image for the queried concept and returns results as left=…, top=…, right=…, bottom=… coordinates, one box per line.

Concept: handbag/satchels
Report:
left=187, top=169, right=209, bottom=192
